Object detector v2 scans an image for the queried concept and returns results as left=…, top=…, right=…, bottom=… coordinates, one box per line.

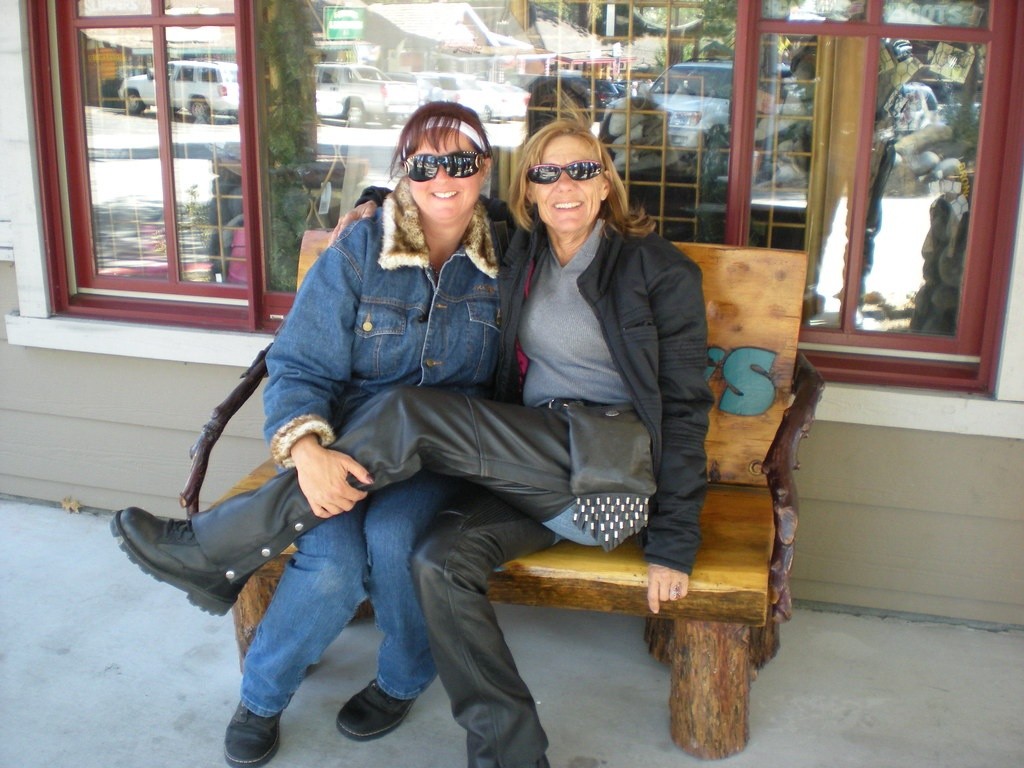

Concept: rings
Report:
left=671, top=586, right=682, bottom=598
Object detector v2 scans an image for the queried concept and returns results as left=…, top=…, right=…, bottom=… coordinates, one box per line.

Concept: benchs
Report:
left=175, top=229, right=824, bottom=760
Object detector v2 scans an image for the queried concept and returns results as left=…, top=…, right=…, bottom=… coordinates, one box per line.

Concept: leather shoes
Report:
left=223, top=701, right=283, bottom=768
left=108, top=506, right=251, bottom=617
left=335, top=677, right=417, bottom=741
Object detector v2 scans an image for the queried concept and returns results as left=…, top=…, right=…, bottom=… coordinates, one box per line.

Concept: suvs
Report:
left=120, top=59, right=239, bottom=124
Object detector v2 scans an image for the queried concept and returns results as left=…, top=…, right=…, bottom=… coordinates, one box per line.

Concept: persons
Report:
left=218, top=100, right=501, bottom=767
left=109, top=120, right=715, bottom=768
left=790, top=9, right=913, bottom=329
left=487, top=74, right=728, bottom=214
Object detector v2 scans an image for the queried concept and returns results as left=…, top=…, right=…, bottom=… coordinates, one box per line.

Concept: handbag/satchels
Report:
left=548, top=399, right=658, bottom=553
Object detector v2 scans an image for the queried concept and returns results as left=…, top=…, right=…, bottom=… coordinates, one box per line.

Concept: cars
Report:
left=879, top=79, right=982, bottom=169
left=316, top=58, right=818, bottom=191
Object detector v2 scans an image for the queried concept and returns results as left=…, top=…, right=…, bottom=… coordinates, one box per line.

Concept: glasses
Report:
left=401, top=150, right=489, bottom=182
left=526, top=161, right=605, bottom=184
